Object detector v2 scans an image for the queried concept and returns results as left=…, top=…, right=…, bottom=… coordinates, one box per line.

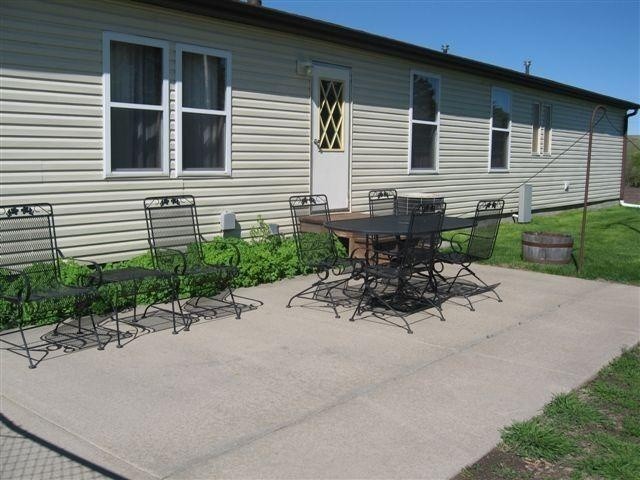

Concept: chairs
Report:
left=285, top=188, right=505, bottom=334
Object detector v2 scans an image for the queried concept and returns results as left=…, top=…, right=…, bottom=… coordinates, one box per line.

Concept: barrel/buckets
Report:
left=522, top=230, right=574, bottom=265
